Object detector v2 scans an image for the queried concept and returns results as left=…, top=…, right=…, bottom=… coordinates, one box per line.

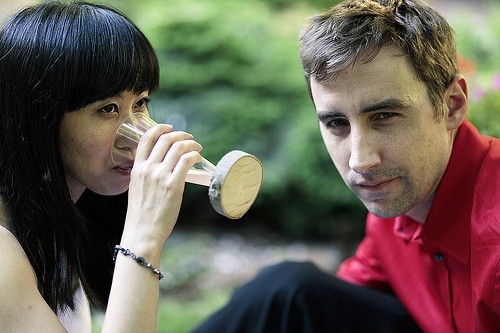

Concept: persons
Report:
left=1, top=0, right=198, bottom=333
left=183, top=0, right=500, bottom=333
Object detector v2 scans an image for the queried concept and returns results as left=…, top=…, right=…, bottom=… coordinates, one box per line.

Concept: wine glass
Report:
left=110, top=112, right=263, bottom=221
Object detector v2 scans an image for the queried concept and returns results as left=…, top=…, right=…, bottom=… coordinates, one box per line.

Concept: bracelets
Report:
left=113, top=245, right=164, bottom=280
